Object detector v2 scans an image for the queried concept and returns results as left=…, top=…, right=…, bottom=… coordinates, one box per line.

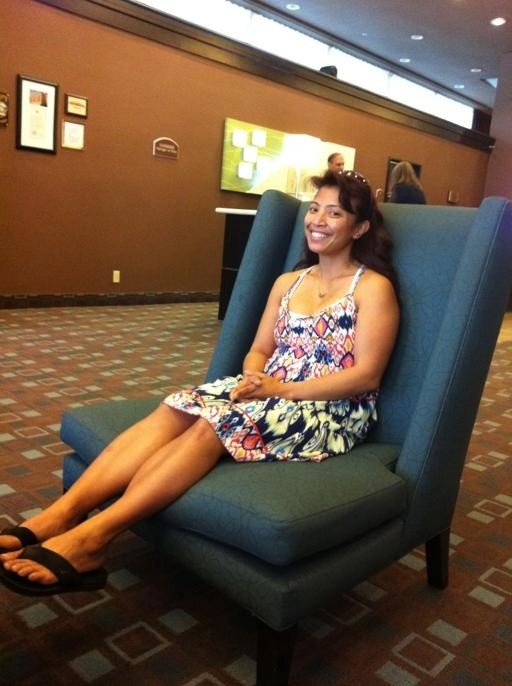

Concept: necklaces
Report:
left=317, top=261, right=352, bottom=298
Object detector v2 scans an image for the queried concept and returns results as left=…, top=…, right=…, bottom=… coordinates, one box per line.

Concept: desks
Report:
left=215, top=207, right=257, bottom=320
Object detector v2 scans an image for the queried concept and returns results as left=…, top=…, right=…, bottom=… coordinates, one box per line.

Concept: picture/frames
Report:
left=17, top=73, right=58, bottom=155
left=64, top=94, right=88, bottom=116
left=61, top=120, right=86, bottom=152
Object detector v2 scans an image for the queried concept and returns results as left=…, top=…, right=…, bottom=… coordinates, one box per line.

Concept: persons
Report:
left=387, top=160, right=427, bottom=204
left=0, top=162, right=403, bottom=598
left=327, top=152, right=345, bottom=174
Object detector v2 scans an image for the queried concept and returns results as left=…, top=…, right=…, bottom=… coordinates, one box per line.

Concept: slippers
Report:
left=0, top=542, right=108, bottom=597
left=0, top=522, right=38, bottom=555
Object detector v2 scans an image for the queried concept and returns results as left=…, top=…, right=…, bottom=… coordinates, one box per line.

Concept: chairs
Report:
left=58, top=189, right=511, bottom=686
